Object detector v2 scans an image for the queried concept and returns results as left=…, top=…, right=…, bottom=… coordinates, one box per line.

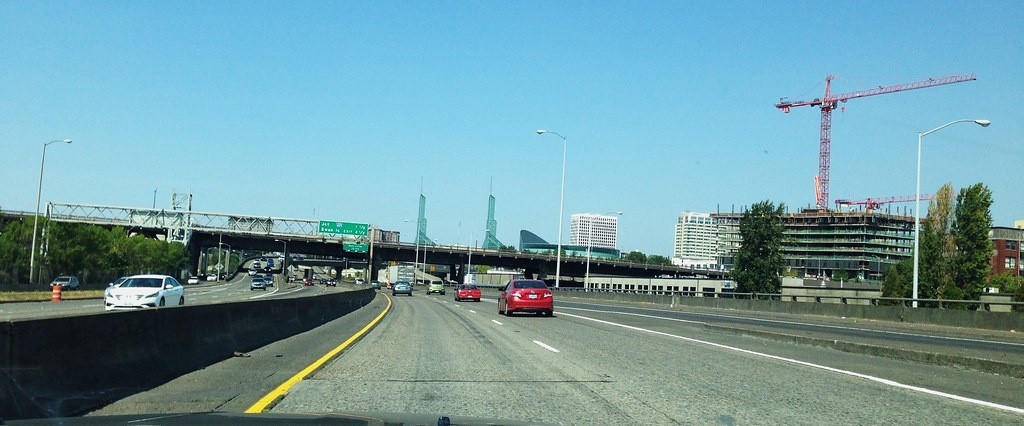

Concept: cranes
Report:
left=774, top=72, right=977, bottom=213
left=834, top=193, right=935, bottom=213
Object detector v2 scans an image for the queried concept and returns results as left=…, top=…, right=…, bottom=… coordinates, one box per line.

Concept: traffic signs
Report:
left=343, top=241, right=368, bottom=253
left=319, top=220, right=369, bottom=236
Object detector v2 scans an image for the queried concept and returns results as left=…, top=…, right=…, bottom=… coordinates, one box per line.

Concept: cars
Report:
left=103, top=274, right=186, bottom=311
left=392, top=281, right=413, bottom=296
left=425, top=280, right=446, bottom=296
left=187, top=276, right=200, bottom=285
left=247, top=255, right=337, bottom=291
left=372, top=280, right=382, bottom=290
left=355, top=278, right=364, bottom=285
left=206, top=271, right=232, bottom=282
left=497, top=278, right=554, bottom=317
left=453, top=284, right=482, bottom=302
left=49, top=275, right=80, bottom=291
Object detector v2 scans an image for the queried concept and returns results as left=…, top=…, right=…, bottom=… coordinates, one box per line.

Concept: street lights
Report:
left=584, top=211, right=624, bottom=292
left=274, top=239, right=286, bottom=274
left=422, top=238, right=437, bottom=284
left=466, top=229, right=490, bottom=284
left=404, top=219, right=420, bottom=284
left=204, top=247, right=217, bottom=279
left=219, top=242, right=231, bottom=279
left=28, top=138, right=73, bottom=284
left=536, top=128, right=568, bottom=291
left=910, top=118, right=993, bottom=307
